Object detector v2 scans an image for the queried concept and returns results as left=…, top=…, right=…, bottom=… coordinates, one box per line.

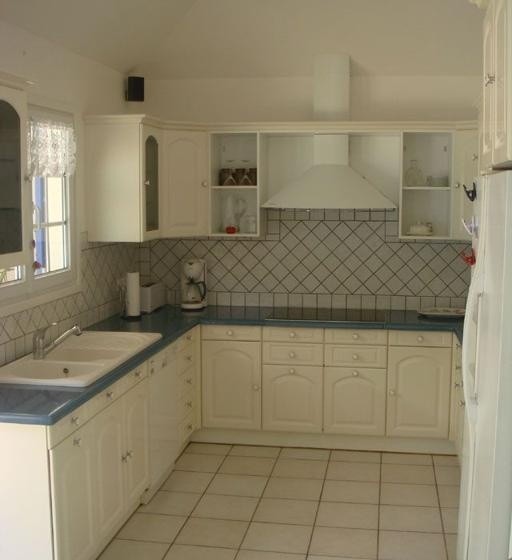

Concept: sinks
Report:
left=45, top=331, right=162, bottom=362
left=0, top=362, right=121, bottom=388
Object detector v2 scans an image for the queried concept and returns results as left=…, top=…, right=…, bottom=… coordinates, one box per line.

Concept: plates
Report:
left=417, top=308, right=465, bottom=317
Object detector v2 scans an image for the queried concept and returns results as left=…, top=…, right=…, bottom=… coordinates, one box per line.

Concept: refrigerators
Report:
left=459, top=172, right=511, bottom=547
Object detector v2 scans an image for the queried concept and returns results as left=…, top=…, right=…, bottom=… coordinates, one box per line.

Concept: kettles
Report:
left=218, top=191, right=247, bottom=232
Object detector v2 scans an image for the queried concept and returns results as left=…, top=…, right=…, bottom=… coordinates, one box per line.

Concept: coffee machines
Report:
left=180, top=258, right=207, bottom=311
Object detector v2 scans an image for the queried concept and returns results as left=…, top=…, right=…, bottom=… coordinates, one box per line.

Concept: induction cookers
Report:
left=266, top=306, right=389, bottom=324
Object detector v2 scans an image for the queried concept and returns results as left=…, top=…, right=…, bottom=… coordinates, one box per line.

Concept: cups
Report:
left=430, top=175, right=449, bottom=187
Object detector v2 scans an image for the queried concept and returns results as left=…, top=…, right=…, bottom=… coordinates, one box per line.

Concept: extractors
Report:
left=260, top=54, right=399, bottom=211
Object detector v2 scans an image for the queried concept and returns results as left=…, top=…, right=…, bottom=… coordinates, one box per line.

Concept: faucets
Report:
left=33, top=322, right=82, bottom=360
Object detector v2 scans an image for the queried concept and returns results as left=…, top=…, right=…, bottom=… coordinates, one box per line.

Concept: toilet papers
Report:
left=127, top=271, right=139, bottom=316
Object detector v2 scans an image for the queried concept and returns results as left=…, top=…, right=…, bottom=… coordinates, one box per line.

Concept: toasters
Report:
left=139, top=281, right=166, bottom=314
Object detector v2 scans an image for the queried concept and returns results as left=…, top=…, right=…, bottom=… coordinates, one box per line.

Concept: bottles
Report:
left=404, top=160, right=426, bottom=186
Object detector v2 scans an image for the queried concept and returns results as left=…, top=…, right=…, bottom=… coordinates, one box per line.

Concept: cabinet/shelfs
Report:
left=163, top=121, right=222, bottom=238
left=209, top=121, right=268, bottom=237
left=201, top=322, right=452, bottom=443
left=147, top=324, right=201, bottom=489
left=452, top=331, right=466, bottom=464
left=0, top=365, right=146, bottom=559
left=0, top=84, right=32, bottom=270
left=82, top=114, right=162, bottom=243
left=480, top=1, right=511, bottom=170
left=397, top=120, right=479, bottom=240
